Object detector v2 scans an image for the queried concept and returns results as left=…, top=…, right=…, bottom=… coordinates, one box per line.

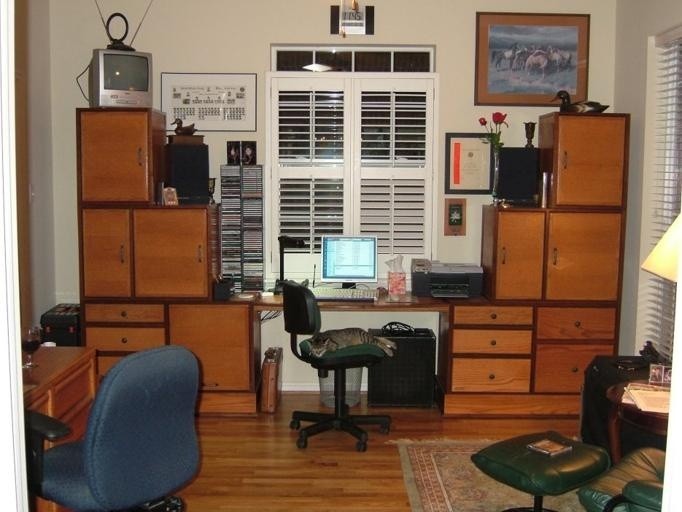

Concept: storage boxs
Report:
left=261, top=346, right=283, bottom=413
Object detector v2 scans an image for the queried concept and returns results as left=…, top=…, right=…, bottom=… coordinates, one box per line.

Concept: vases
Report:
left=491, top=154, right=508, bottom=206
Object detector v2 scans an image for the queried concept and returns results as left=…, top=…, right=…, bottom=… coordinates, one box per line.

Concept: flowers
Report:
left=477, top=112, right=508, bottom=153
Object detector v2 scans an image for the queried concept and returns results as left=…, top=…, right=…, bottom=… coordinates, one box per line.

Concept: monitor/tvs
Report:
left=320, top=234, right=378, bottom=288
left=87, top=48, right=157, bottom=111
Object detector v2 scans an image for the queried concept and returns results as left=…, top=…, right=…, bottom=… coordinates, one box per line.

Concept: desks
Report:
left=24, top=344, right=97, bottom=512
left=252, top=290, right=449, bottom=413
left=605, top=375, right=672, bottom=469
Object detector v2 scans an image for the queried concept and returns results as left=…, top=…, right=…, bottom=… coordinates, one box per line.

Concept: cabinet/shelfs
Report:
left=480, top=205, right=548, bottom=304
left=78, top=299, right=166, bottom=383
left=131, top=203, right=224, bottom=299
left=436, top=300, right=534, bottom=393
left=544, top=209, right=629, bottom=304
left=531, top=304, right=623, bottom=397
left=536, top=112, right=631, bottom=209
left=77, top=107, right=166, bottom=206
left=167, top=291, right=262, bottom=392
left=76, top=205, right=132, bottom=302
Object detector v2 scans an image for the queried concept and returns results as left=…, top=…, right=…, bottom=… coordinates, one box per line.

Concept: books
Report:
left=527, top=437, right=571, bottom=456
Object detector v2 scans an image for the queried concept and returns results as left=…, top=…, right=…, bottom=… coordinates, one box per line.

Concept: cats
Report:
left=307, top=327, right=398, bottom=358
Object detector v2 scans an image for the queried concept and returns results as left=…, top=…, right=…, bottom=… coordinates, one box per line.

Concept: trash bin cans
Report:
left=318, top=366, right=363, bottom=408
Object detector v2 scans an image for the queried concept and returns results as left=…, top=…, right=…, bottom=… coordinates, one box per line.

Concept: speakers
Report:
left=161, top=143, right=210, bottom=203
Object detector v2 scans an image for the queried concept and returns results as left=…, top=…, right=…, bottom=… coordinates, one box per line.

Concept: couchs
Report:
left=578, top=446, right=666, bottom=512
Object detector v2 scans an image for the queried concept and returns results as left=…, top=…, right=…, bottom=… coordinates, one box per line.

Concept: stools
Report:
left=470, top=429, right=611, bottom=512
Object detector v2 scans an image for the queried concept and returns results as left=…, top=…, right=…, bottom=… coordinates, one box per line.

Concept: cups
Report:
left=22, top=325, right=43, bottom=370
left=523, top=121, right=537, bottom=147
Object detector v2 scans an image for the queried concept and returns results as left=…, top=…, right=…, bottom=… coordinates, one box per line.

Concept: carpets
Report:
left=393, top=439, right=590, bottom=512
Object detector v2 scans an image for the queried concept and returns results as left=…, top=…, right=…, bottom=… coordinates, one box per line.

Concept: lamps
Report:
left=640, top=213, right=682, bottom=284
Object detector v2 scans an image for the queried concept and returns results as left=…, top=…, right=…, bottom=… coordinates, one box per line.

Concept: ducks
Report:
left=170, top=117, right=197, bottom=136
left=551, top=89, right=611, bottom=116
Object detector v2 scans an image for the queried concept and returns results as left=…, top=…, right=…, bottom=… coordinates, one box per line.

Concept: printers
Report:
left=410, top=258, right=485, bottom=299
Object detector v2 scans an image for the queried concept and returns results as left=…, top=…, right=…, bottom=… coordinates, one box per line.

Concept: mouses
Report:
left=390, top=293, right=401, bottom=302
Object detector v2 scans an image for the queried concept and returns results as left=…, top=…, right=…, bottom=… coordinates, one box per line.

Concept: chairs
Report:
left=282, top=281, right=398, bottom=452
left=25, top=345, right=203, bottom=512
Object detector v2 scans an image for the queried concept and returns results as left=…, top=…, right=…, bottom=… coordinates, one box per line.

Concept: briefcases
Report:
left=259, top=346, right=284, bottom=413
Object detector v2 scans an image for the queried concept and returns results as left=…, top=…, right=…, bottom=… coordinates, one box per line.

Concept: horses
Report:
left=491, top=42, right=520, bottom=73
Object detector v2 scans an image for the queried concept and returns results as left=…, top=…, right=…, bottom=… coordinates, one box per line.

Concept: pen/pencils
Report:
left=211, top=271, right=224, bottom=282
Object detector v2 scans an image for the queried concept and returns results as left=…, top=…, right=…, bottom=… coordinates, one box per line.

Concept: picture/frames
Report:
left=473, top=11, right=591, bottom=106
left=160, top=72, right=258, bottom=133
left=446, top=131, right=497, bottom=194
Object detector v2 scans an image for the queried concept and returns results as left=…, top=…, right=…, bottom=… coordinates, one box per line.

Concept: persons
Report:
left=650, top=367, right=661, bottom=382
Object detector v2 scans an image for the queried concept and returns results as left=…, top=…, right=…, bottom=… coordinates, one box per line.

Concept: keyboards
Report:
left=311, top=287, right=380, bottom=302
